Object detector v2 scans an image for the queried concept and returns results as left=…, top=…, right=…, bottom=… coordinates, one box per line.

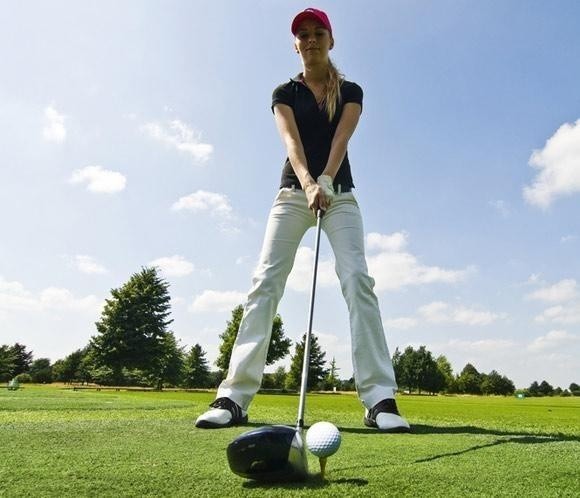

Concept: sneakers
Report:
left=364, top=399, right=410, bottom=430
left=196, top=398, right=247, bottom=427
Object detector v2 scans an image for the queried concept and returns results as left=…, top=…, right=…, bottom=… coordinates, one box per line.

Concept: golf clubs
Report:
left=228, top=209, right=324, bottom=481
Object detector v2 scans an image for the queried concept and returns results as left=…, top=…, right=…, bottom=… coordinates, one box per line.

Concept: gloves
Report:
left=317, top=175, right=335, bottom=204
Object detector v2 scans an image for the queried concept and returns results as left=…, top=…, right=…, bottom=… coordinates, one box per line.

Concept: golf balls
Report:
left=306, top=421, right=341, bottom=458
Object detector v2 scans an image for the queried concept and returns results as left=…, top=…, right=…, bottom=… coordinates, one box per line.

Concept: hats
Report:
left=292, top=8, right=331, bottom=35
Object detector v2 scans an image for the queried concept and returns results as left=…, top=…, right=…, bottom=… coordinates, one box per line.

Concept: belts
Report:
left=284, top=182, right=351, bottom=191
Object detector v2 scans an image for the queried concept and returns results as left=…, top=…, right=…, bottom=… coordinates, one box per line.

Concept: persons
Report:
left=196, top=8, right=411, bottom=431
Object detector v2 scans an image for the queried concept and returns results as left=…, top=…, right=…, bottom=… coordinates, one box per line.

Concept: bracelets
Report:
left=316, top=175, right=333, bottom=188
